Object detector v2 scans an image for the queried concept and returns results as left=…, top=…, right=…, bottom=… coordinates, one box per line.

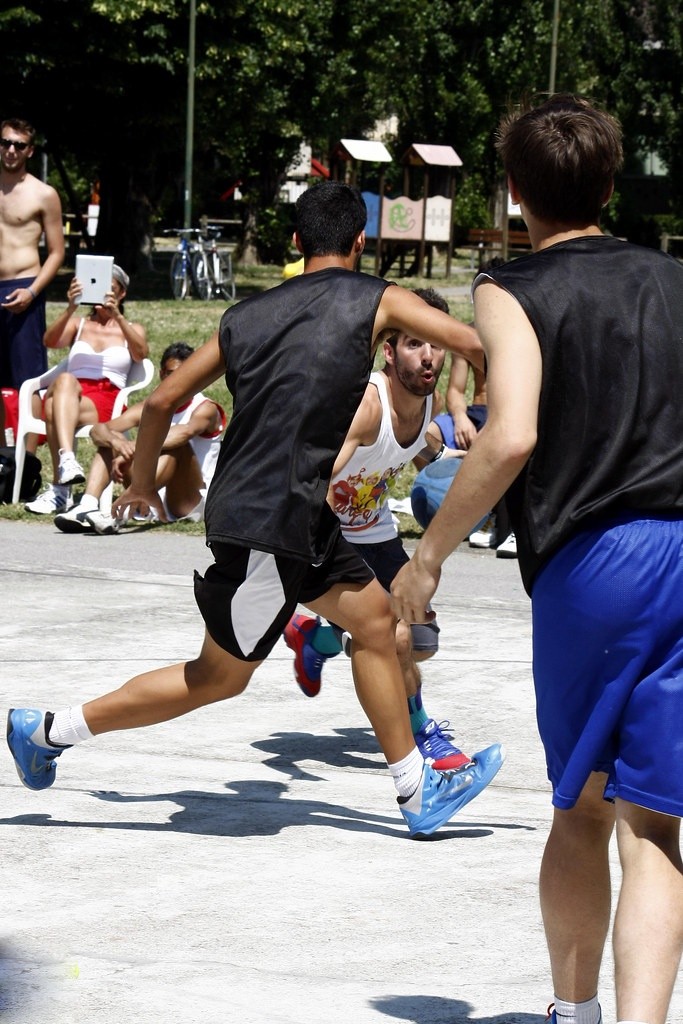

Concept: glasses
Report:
left=0, top=138, right=32, bottom=151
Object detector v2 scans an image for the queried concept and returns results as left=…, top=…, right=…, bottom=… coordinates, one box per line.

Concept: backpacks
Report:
left=0, top=446, right=42, bottom=505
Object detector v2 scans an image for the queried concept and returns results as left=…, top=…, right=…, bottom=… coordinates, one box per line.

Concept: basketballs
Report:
left=411, top=457, right=489, bottom=538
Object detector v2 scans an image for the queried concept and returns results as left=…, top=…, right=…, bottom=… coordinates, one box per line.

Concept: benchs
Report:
left=462, top=230, right=533, bottom=270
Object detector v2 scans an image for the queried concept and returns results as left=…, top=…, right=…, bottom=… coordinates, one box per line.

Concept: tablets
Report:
left=74, top=255, right=114, bottom=305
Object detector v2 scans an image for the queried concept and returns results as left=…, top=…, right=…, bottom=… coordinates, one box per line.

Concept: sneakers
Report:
left=7, top=709, right=73, bottom=791
left=54, top=503, right=126, bottom=535
left=414, top=718, right=472, bottom=770
left=57, top=448, right=86, bottom=486
left=397, top=743, right=507, bottom=840
left=496, top=531, right=518, bottom=558
left=283, top=612, right=340, bottom=697
left=544, top=1003, right=602, bottom=1024
left=469, top=512, right=498, bottom=548
left=24, top=483, right=74, bottom=515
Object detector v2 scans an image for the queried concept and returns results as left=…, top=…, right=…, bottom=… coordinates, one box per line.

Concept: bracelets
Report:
left=26, top=287, right=36, bottom=298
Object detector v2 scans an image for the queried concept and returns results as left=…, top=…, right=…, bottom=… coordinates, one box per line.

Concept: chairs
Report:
left=11, top=355, right=155, bottom=512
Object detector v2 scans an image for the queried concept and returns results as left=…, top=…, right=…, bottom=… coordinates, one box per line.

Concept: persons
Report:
left=23, top=263, right=148, bottom=515
left=76, top=192, right=102, bottom=245
left=0, top=119, right=65, bottom=503
left=279, top=288, right=472, bottom=774
left=411, top=321, right=517, bottom=560
left=381, top=94, right=683, bottom=1024
left=53, top=341, right=226, bottom=535
left=3, top=180, right=507, bottom=843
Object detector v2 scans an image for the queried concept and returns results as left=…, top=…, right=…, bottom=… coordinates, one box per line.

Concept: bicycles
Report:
left=163, top=224, right=236, bottom=301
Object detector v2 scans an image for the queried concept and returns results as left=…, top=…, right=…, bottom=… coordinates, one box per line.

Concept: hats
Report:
left=113, top=264, right=130, bottom=290
